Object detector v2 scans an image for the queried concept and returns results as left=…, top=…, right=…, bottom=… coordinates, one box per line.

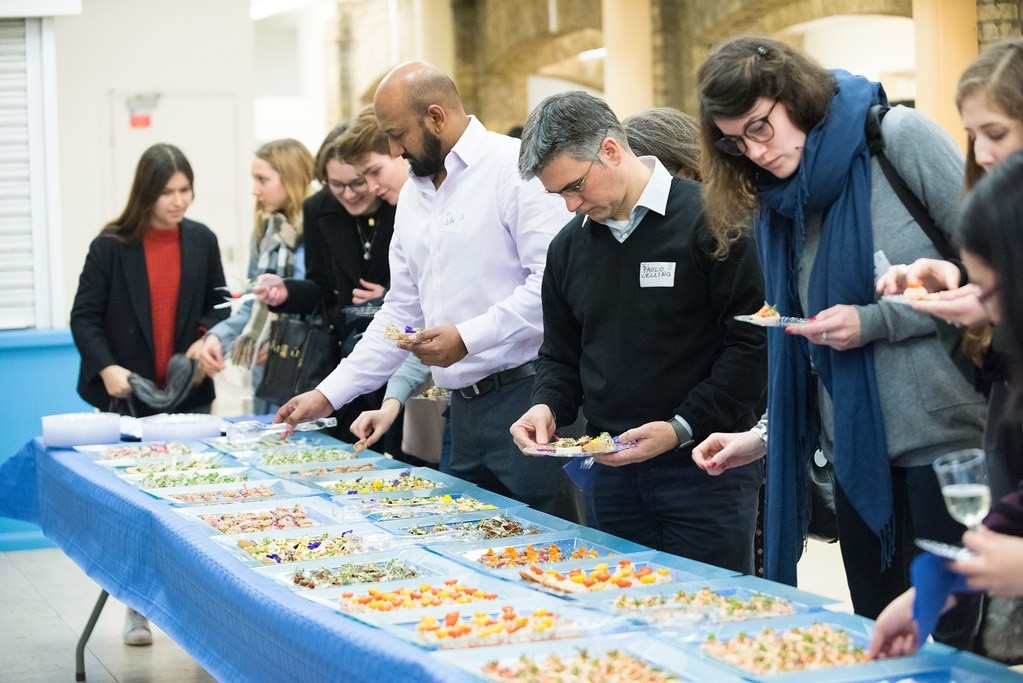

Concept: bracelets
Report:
left=752, top=423, right=768, bottom=442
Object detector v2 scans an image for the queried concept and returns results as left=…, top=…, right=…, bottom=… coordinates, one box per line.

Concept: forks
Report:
left=579, top=457, right=597, bottom=472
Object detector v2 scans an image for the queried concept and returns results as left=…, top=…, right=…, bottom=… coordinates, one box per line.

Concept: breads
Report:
left=582, top=441, right=606, bottom=452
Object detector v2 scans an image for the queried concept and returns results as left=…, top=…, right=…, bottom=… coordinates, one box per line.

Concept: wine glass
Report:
left=932, top=448, right=993, bottom=533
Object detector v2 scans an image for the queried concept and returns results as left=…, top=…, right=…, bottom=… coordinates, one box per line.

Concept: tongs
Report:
left=212, top=280, right=283, bottom=309
left=226, top=417, right=337, bottom=443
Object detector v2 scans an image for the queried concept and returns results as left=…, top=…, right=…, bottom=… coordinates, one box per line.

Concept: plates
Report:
left=735, top=316, right=810, bottom=327
left=915, top=537, right=980, bottom=566
left=361, top=330, right=429, bottom=346
left=881, top=294, right=916, bottom=306
left=522, top=443, right=627, bottom=456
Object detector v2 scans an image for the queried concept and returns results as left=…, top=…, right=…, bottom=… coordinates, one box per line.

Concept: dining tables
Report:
left=0, top=410, right=1023, bottom=682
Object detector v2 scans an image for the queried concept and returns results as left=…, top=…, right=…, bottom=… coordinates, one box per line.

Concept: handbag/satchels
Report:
left=254, top=284, right=344, bottom=406
left=402, top=374, right=452, bottom=464
left=803, top=455, right=839, bottom=545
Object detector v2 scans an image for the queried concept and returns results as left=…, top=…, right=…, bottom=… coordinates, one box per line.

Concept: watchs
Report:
left=668, top=418, right=695, bottom=454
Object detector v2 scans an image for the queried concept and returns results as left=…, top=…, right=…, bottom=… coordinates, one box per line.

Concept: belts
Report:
left=459, top=359, right=543, bottom=399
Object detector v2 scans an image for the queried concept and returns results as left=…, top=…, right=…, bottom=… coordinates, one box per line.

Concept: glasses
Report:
left=976, top=283, right=1000, bottom=318
left=544, top=127, right=610, bottom=199
left=714, top=97, right=780, bottom=156
left=326, top=178, right=369, bottom=195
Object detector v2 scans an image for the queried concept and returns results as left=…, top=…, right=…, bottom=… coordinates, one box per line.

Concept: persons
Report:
left=509, top=90, right=766, bottom=578
left=274, top=61, right=576, bottom=502
left=198, top=138, right=320, bottom=416
left=691, top=36, right=990, bottom=623
left=254, top=125, right=387, bottom=329
left=69, top=142, right=232, bottom=646
left=869, top=153, right=1023, bottom=657
left=877, top=43, right=1023, bottom=666
left=623, top=108, right=766, bottom=577
left=338, top=105, right=440, bottom=473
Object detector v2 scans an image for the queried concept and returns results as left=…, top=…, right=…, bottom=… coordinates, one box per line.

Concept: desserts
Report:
left=341, top=544, right=671, bottom=642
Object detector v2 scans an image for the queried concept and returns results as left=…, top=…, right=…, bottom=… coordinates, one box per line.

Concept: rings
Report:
left=821, top=331, right=827, bottom=343
